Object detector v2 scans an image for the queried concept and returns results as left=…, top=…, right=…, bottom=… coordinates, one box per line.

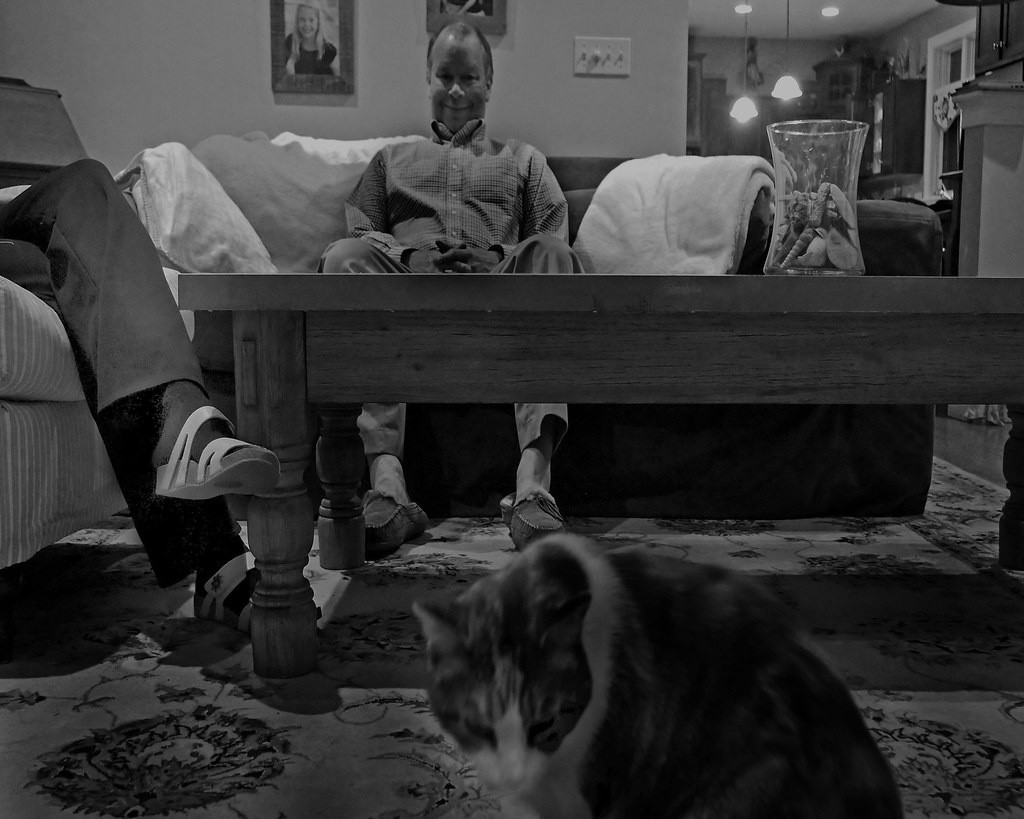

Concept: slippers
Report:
left=154, top=405, right=281, bottom=500
left=193, top=550, right=322, bottom=633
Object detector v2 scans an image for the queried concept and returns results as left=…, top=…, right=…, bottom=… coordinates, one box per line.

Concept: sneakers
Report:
left=360, top=489, right=429, bottom=560
left=499, top=486, right=567, bottom=554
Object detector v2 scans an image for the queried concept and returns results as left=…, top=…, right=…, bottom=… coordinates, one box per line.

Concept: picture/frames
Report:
left=426, top=1, right=508, bottom=37
left=269, top=0, right=355, bottom=96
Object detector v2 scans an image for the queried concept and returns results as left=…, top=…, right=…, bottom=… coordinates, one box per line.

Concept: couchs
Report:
left=113, top=157, right=946, bottom=517
left=1, top=263, right=194, bottom=661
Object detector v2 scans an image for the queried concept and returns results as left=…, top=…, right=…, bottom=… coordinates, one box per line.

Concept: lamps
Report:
left=729, top=0, right=758, bottom=123
left=771, top=0, right=803, bottom=101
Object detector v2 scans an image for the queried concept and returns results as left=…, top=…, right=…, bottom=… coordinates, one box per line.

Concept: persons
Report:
left=280, top=0, right=337, bottom=77
left=317, top=24, right=587, bottom=554
left=438, top=0, right=488, bottom=17
left=1, top=158, right=322, bottom=631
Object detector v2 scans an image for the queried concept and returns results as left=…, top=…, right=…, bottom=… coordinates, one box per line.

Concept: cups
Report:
left=763, top=119, right=869, bottom=276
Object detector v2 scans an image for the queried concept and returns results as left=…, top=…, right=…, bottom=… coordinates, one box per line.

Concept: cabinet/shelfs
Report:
left=812, top=61, right=923, bottom=174
left=974, top=0, right=1024, bottom=75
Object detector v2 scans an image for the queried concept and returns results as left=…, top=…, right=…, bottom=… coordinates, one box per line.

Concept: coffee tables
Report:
left=173, top=268, right=1023, bottom=671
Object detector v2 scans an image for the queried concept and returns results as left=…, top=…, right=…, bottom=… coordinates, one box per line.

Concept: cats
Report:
left=410, top=533, right=906, bottom=819
